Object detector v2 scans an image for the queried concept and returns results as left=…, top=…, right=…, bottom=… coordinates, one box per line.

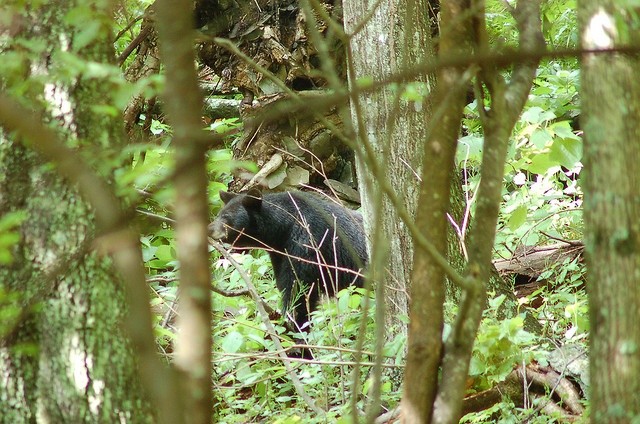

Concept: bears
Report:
left=204, top=185, right=368, bottom=336
